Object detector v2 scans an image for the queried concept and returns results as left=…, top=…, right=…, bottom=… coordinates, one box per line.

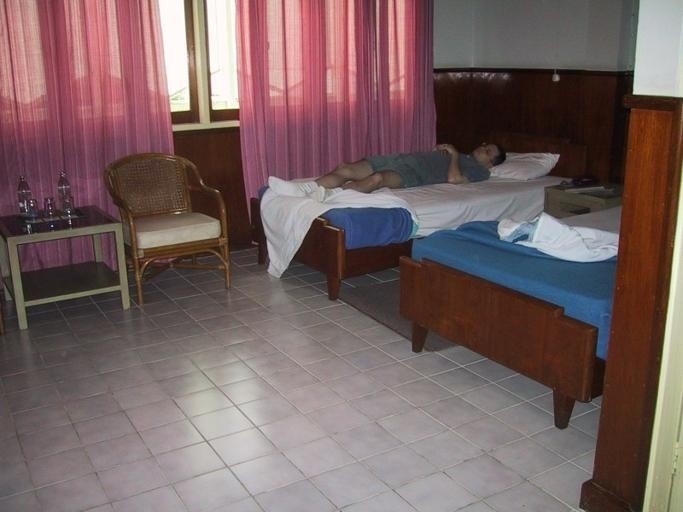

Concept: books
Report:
left=555, top=182, right=606, bottom=194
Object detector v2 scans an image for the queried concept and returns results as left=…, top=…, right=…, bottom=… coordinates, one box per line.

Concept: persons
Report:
left=268, top=141, right=506, bottom=203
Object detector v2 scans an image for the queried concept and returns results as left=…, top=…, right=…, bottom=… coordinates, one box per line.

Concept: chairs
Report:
left=102, top=152, right=232, bottom=307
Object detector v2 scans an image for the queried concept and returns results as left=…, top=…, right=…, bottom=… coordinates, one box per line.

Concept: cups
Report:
left=62, top=196, right=74, bottom=219
left=43, top=198, right=55, bottom=221
left=25, top=199, right=38, bottom=222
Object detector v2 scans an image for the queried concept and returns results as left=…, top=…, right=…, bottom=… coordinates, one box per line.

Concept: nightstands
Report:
left=544, top=184, right=623, bottom=220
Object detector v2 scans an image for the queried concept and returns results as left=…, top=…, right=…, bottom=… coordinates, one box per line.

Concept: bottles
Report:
left=58, top=170, right=70, bottom=203
left=17, top=176, right=33, bottom=216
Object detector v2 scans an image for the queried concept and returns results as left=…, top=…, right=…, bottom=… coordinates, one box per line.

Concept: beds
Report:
left=396, top=205, right=621, bottom=429
left=249, top=128, right=588, bottom=301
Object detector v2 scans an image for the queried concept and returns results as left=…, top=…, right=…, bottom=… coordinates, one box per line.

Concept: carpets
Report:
left=339, top=279, right=458, bottom=352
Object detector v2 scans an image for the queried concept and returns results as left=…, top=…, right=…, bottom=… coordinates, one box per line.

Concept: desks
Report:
left=1, top=204, right=130, bottom=331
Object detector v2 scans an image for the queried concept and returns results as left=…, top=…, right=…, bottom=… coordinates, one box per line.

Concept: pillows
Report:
left=491, top=152, right=562, bottom=183
